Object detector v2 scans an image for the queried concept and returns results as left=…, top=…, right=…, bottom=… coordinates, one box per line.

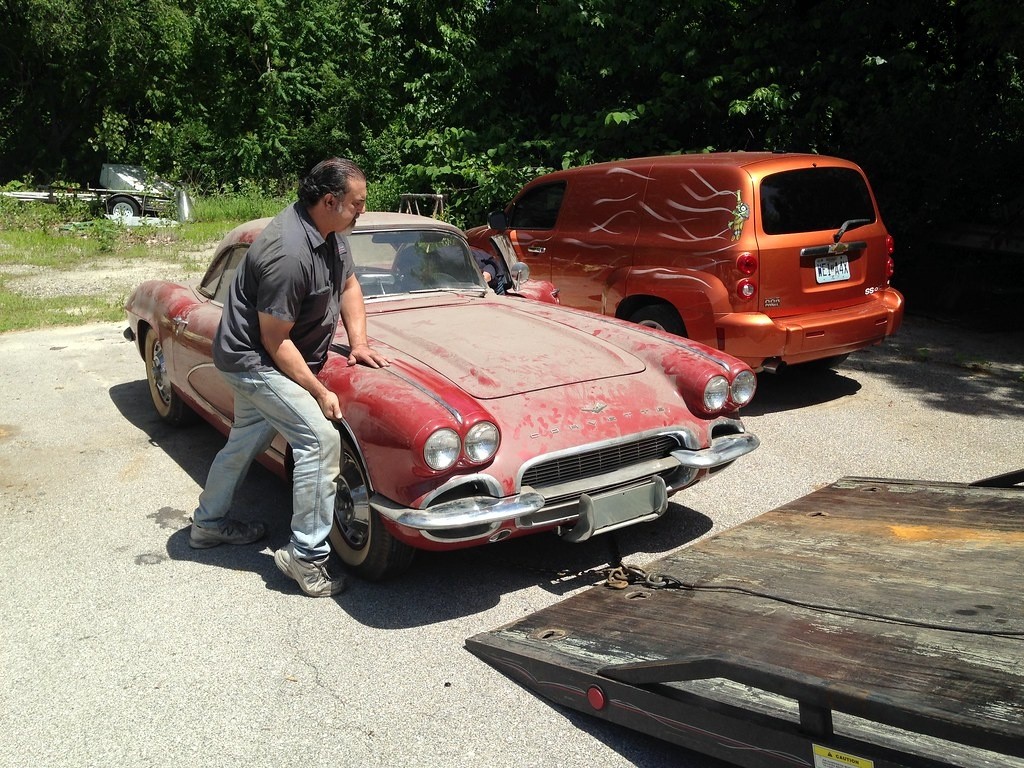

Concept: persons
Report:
left=190, top=157, right=392, bottom=596
left=391, top=245, right=505, bottom=295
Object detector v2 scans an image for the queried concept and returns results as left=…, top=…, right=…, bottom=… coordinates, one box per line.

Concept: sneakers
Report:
left=188, top=515, right=267, bottom=549
left=273, top=543, right=346, bottom=597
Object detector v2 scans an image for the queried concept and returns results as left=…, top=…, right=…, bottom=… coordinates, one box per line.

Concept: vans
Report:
left=450, top=153, right=904, bottom=382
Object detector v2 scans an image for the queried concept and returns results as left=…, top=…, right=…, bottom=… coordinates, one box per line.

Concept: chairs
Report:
left=392, top=245, right=439, bottom=293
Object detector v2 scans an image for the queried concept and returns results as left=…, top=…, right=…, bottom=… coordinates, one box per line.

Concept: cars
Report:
left=124, top=209, right=761, bottom=574
